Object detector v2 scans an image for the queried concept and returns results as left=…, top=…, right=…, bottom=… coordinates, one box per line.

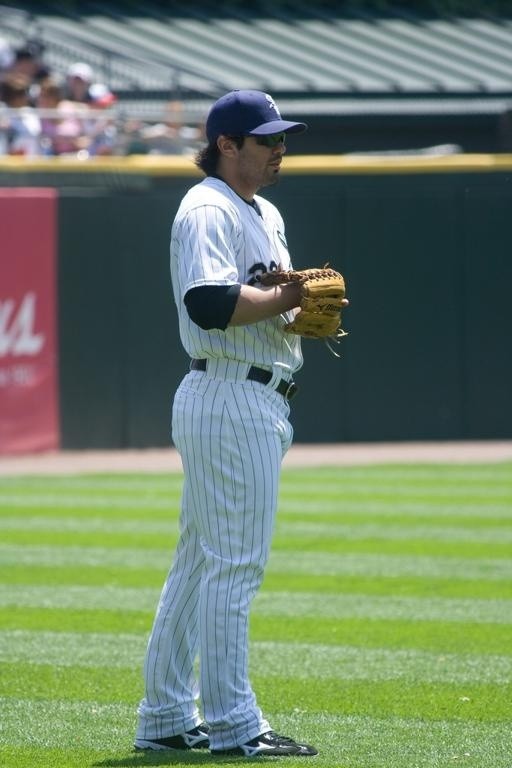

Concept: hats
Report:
left=206, top=89, right=308, bottom=144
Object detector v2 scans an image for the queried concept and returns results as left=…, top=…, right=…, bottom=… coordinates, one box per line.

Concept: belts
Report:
left=191, top=360, right=299, bottom=402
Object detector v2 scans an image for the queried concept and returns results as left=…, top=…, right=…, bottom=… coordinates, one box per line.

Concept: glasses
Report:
left=256, top=132, right=286, bottom=148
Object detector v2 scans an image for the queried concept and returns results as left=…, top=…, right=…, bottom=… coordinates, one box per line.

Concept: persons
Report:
left=132, top=88, right=318, bottom=756
left=0, top=38, right=208, bottom=159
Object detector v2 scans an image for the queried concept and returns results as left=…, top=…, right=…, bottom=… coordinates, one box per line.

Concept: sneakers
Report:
left=211, top=731, right=320, bottom=758
left=134, top=724, right=210, bottom=751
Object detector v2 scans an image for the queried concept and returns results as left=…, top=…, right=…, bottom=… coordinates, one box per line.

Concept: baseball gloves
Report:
left=258, top=266, right=345, bottom=339
